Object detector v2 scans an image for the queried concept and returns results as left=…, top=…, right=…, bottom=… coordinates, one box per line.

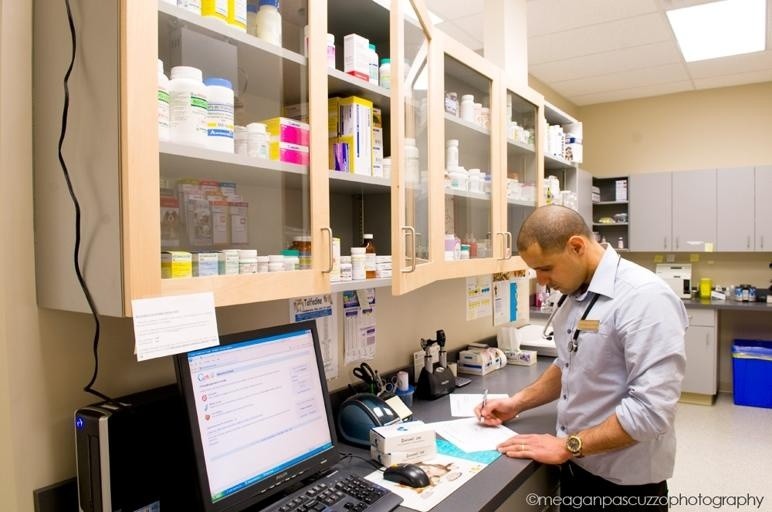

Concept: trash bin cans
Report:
left=730, top=339, right=772, bottom=408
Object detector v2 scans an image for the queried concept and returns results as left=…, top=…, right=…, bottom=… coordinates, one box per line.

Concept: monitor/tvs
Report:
left=173, top=320, right=342, bottom=512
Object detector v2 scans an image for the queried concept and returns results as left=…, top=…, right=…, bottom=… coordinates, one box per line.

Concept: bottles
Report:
left=381, top=157, right=391, bottom=179
left=699, top=278, right=772, bottom=303
left=444, top=89, right=490, bottom=129
left=367, top=43, right=379, bottom=86
left=504, top=170, right=578, bottom=211
left=543, top=118, right=583, bottom=165
left=403, top=136, right=420, bottom=185
left=592, top=213, right=629, bottom=249
left=379, top=57, right=392, bottom=89
left=329, top=232, right=377, bottom=282
left=444, top=230, right=492, bottom=260
left=239, top=237, right=311, bottom=273
left=505, top=95, right=534, bottom=146
left=177, top=0, right=283, bottom=48
left=325, top=32, right=337, bottom=70
left=156, top=58, right=270, bottom=162
left=444, top=139, right=491, bottom=197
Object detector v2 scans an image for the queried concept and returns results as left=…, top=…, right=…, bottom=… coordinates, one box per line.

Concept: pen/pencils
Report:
left=375, top=370, right=382, bottom=385
left=481, top=388, right=489, bottom=426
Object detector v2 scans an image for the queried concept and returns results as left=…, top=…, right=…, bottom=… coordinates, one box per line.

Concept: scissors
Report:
left=377, top=375, right=399, bottom=395
left=353, top=362, right=377, bottom=384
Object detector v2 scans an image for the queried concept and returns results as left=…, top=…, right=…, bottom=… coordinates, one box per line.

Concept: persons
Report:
left=474, top=204, right=689, bottom=511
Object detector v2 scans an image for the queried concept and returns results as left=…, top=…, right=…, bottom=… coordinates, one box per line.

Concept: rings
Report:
left=521, top=438, right=525, bottom=445
left=520, top=444, right=525, bottom=450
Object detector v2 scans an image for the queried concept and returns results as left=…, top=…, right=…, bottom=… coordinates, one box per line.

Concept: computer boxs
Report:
left=74, top=382, right=204, bottom=511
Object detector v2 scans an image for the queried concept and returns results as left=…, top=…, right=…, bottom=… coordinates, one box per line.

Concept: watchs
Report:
left=566, top=432, right=585, bottom=458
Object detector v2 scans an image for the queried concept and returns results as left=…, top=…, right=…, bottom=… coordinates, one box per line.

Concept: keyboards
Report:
left=259, top=466, right=404, bottom=512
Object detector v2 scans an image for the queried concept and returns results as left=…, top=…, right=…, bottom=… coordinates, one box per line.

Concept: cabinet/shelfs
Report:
left=674, top=308, right=719, bottom=405
left=33, top=0, right=435, bottom=321
left=435, top=27, right=582, bottom=284
left=577, top=169, right=772, bottom=255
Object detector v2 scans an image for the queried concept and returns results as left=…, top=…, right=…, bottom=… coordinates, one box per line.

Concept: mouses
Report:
left=384, top=463, right=429, bottom=487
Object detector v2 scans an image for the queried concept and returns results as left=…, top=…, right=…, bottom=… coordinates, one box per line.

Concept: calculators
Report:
left=455, top=377, right=472, bottom=388
